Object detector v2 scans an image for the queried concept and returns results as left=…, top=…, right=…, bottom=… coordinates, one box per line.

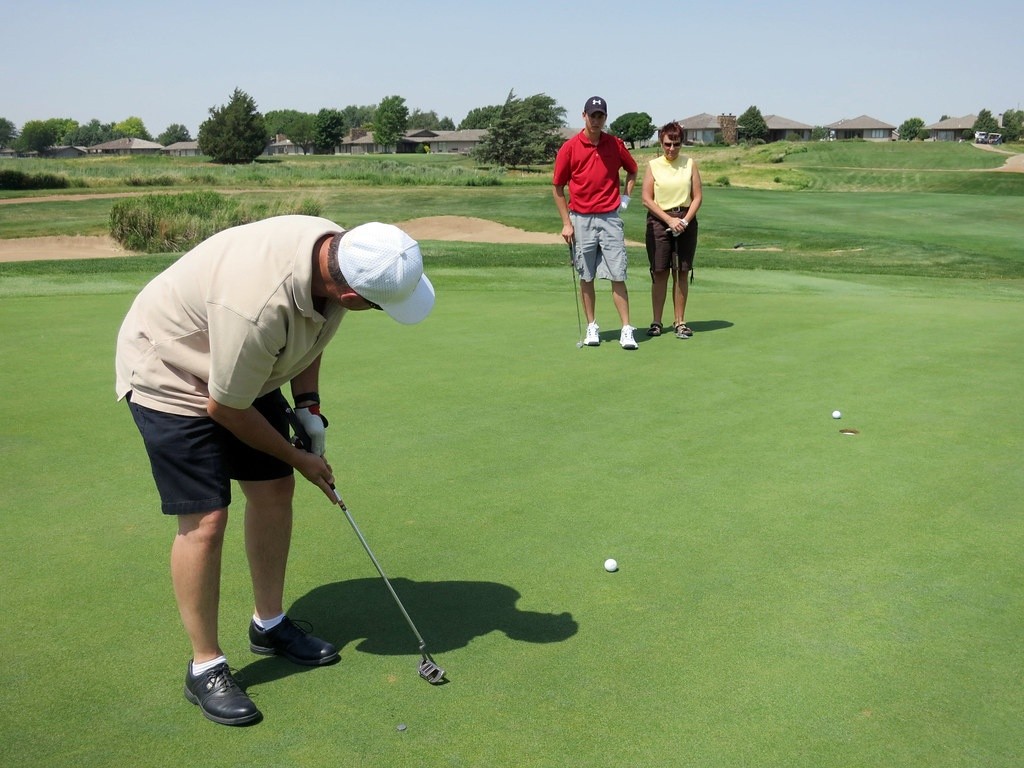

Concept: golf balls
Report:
left=605, top=559, right=617, bottom=572
left=832, top=411, right=841, bottom=419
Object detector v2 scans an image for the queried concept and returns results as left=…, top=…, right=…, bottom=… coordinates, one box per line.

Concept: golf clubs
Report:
left=569, top=241, right=583, bottom=348
left=675, top=237, right=689, bottom=339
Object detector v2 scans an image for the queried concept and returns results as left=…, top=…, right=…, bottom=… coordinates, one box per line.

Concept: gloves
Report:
left=666, top=219, right=689, bottom=237
left=292, top=403, right=329, bottom=464
left=619, top=195, right=631, bottom=213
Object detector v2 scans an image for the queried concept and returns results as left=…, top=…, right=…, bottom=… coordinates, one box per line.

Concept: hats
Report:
left=338, top=222, right=436, bottom=324
left=584, top=96, right=607, bottom=116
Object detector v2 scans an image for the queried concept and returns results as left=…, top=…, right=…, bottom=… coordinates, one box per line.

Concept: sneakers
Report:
left=620, top=325, right=638, bottom=349
left=584, top=319, right=599, bottom=346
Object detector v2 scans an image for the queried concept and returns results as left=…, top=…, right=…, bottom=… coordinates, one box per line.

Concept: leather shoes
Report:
left=248, top=615, right=338, bottom=667
left=184, top=659, right=261, bottom=725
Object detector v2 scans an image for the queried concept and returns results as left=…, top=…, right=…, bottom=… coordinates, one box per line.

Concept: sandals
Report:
left=673, top=321, right=693, bottom=335
left=647, top=322, right=664, bottom=336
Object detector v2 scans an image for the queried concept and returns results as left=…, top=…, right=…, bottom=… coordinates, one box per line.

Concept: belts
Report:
left=671, top=206, right=690, bottom=212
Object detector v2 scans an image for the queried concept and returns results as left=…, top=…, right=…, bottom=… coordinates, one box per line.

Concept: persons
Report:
left=552, top=96, right=639, bottom=350
left=640, top=119, right=702, bottom=338
left=114, top=215, right=436, bottom=726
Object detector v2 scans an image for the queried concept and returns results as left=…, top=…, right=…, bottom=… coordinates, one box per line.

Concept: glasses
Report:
left=665, top=142, right=680, bottom=147
left=364, top=298, right=383, bottom=312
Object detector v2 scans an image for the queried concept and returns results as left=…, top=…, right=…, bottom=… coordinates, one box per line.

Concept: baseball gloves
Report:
left=284, top=407, right=446, bottom=685
